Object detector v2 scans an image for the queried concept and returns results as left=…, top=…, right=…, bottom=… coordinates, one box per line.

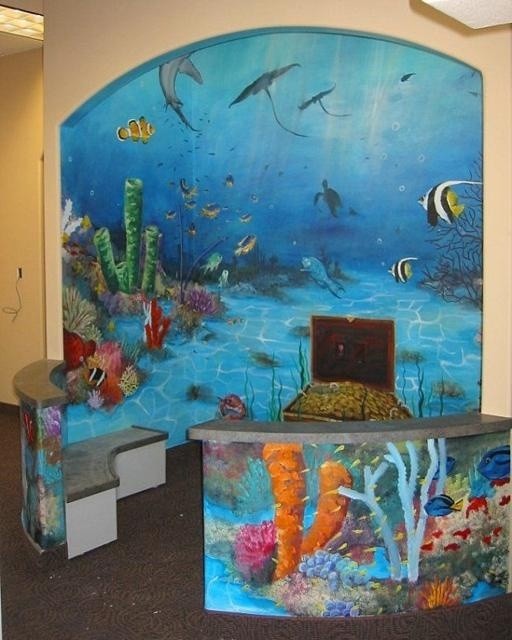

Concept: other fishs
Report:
left=476, top=443, right=511, bottom=481
left=268, top=439, right=427, bottom=617
left=421, top=494, right=464, bottom=519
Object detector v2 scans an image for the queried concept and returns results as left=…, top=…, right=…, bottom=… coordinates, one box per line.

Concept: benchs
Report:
left=61, top=425, right=170, bottom=561
